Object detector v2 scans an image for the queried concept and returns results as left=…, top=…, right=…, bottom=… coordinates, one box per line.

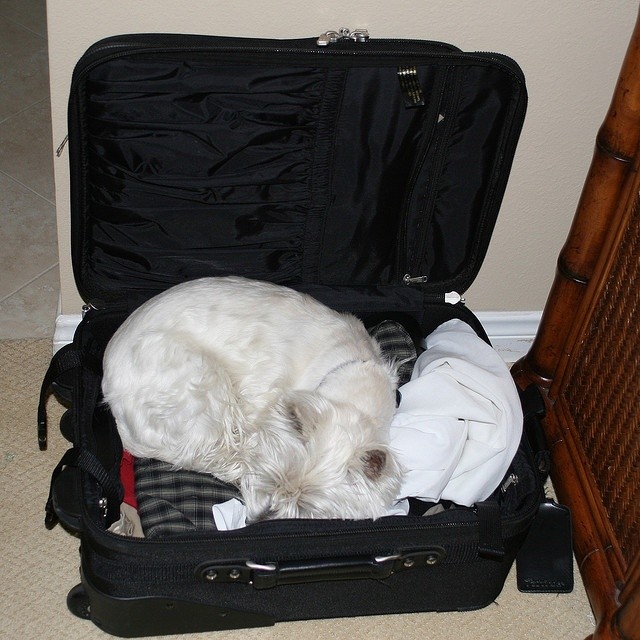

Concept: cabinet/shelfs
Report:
left=513, top=8, right=639, bottom=640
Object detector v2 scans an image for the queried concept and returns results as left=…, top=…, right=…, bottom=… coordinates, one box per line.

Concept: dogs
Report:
left=102, top=276, right=402, bottom=524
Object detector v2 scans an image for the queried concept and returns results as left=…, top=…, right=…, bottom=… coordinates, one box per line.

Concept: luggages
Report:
left=38, top=27, right=543, bottom=637
